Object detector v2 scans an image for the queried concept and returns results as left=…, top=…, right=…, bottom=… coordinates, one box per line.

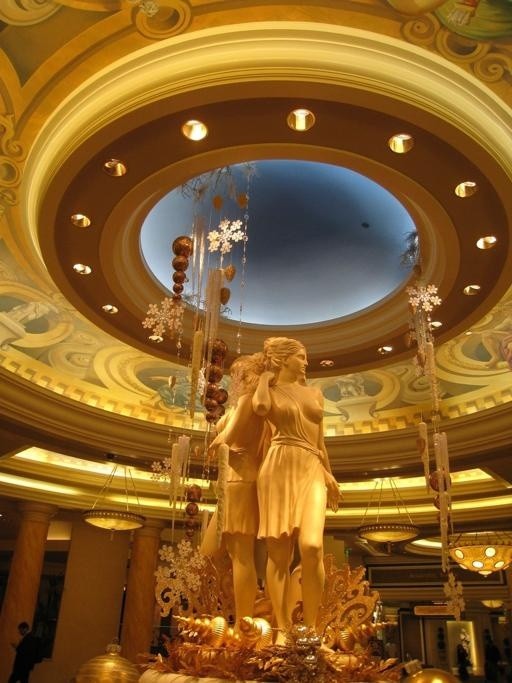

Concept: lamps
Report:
left=81, top=455, right=145, bottom=543
left=445, top=532, right=510, bottom=580
left=356, top=477, right=422, bottom=554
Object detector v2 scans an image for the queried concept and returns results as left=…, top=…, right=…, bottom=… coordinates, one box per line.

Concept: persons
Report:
left=202, top=349, right=266, bottom=639
left=454, top=641, right=470, bottom=682
left=250, top=332, right=331, bottom=642
left=8, top=620, right=36, bottom=682
left=483, top=633, right=500, bottom=682
